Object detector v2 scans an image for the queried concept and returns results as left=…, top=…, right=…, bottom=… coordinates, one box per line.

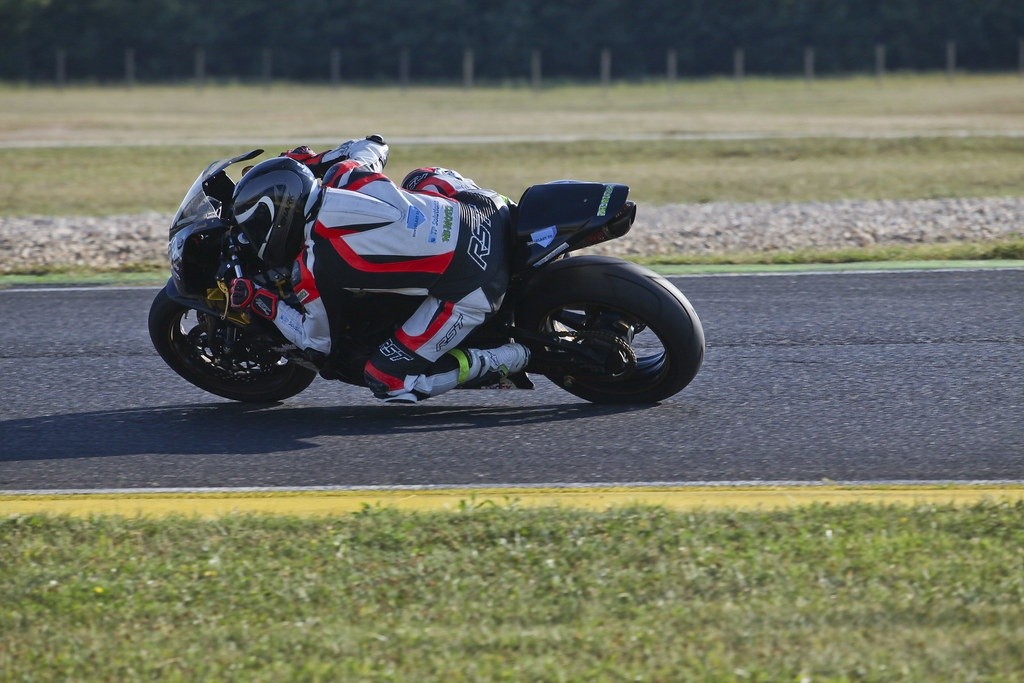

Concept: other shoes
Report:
left=482, top=342, right=531, bottom=377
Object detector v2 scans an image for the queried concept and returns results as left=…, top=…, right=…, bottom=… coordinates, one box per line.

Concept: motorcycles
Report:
left=147, top=149, right=707, bottom=405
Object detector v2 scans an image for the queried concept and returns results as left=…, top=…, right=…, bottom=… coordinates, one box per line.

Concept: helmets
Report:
left=231, top=157, right=322, bottom=269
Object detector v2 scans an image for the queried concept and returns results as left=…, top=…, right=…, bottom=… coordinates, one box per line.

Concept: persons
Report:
left=209, top=134, right=532, bottom=402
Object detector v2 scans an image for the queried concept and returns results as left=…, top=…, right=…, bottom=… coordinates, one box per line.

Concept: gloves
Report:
left=229, top=277, right=277, bottom=321
left=284, top=145, right=332, bottom=178
